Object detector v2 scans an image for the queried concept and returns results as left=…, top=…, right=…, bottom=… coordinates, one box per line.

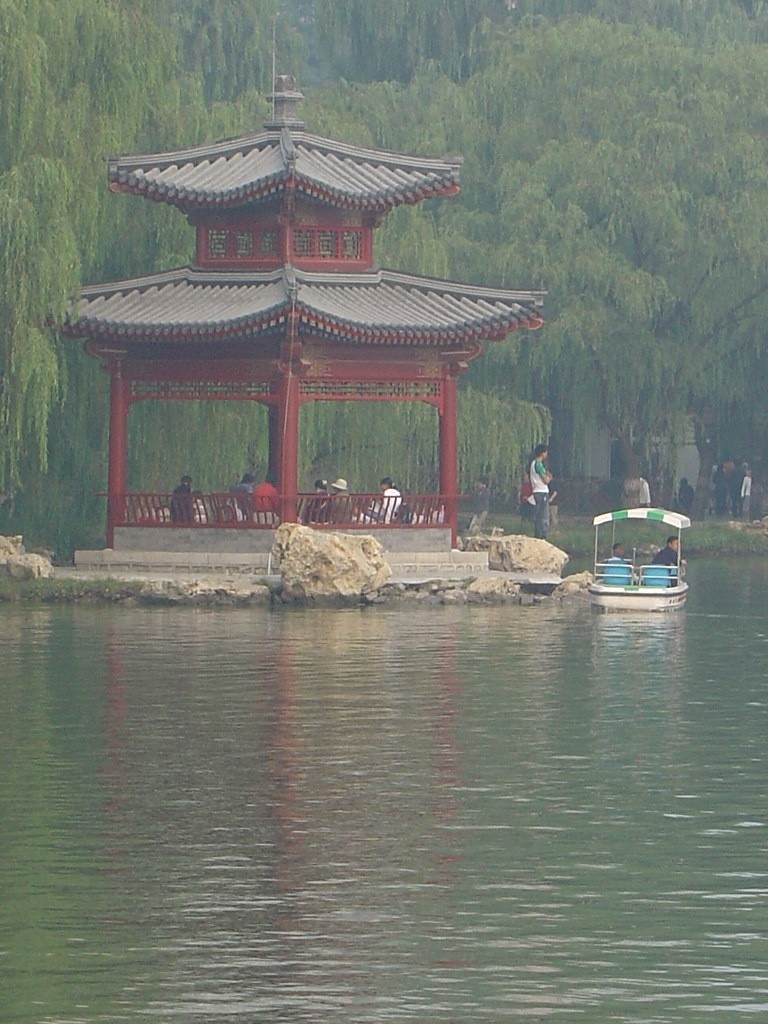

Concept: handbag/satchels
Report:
left=527, top=495, right=536, bottom=506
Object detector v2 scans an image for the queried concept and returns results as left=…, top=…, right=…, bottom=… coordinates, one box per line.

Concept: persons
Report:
left=379, top=477, right=402, bottom=524
left=235, top=471, right=279, bottom=516
left=473, top=479, right=491, bottom=527
left=614, top=543, right=627, bottom=558
left=529, top=444, right=553, bottom=539
left=639, top=474, right=651, bottom=508
left=304, top=478, right=352, bottom=523
left=520, top=472, right=535, bottom=521
left=170, top=476, right=193, bottom=523
left=548, top=472, right=561, bottom=524
left=678, top=478, right=695, bottom=509
left=709, top=456, right=768, bottom=520
left=652, top=536, right=687, bottom=586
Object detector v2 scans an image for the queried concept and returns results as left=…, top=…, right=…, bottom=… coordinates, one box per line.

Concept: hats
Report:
left=331, top=479, right=348, bottom=490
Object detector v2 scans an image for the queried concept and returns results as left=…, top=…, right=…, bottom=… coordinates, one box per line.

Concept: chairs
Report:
left=642, top=564, right=672, bottom=588
left=604, top=560, right=636, bottom=585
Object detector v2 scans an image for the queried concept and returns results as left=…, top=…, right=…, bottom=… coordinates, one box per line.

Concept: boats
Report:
left=588, top=508, right=692, bottom=614
